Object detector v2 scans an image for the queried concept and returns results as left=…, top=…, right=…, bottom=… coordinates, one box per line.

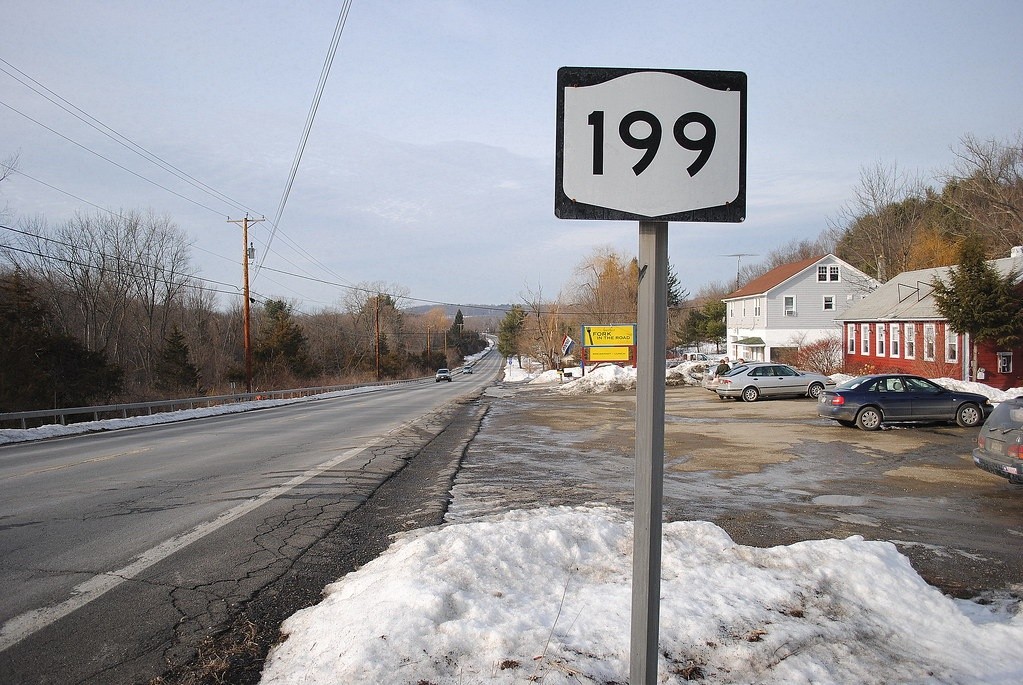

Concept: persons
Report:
left=715, top=359, right=732, bottom=399
left=724, top=357, right=734, bottom=369
left=738, top=359, right=744, bottom=364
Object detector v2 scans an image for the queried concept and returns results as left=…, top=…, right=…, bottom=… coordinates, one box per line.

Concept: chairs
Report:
left=893, top=382, right=902, bottom=392
left=878, top=383, right=887, bottom=392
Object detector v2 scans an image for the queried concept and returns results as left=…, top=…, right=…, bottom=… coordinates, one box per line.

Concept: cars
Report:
left=818, top=372, right=994, bottom=433
left=701, top=363, right=837, bottom=403
left=462, top=366, right=472, bottom=374
left=973, top=395, right=1023, bottom=486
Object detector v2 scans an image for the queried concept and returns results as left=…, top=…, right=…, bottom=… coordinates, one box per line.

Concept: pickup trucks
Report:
left=664, top=353, right=710, bottom=369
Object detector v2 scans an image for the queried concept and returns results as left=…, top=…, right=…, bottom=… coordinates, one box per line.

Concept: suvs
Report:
left=435, top=369, right=452, bottom=382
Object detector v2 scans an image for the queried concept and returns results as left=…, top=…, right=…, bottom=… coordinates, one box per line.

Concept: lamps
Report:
left=883, top=330, right=888, bottom=334
left=868, top=329, right=872, bottom=334
left=854, top=329, right=857, bottom=333
